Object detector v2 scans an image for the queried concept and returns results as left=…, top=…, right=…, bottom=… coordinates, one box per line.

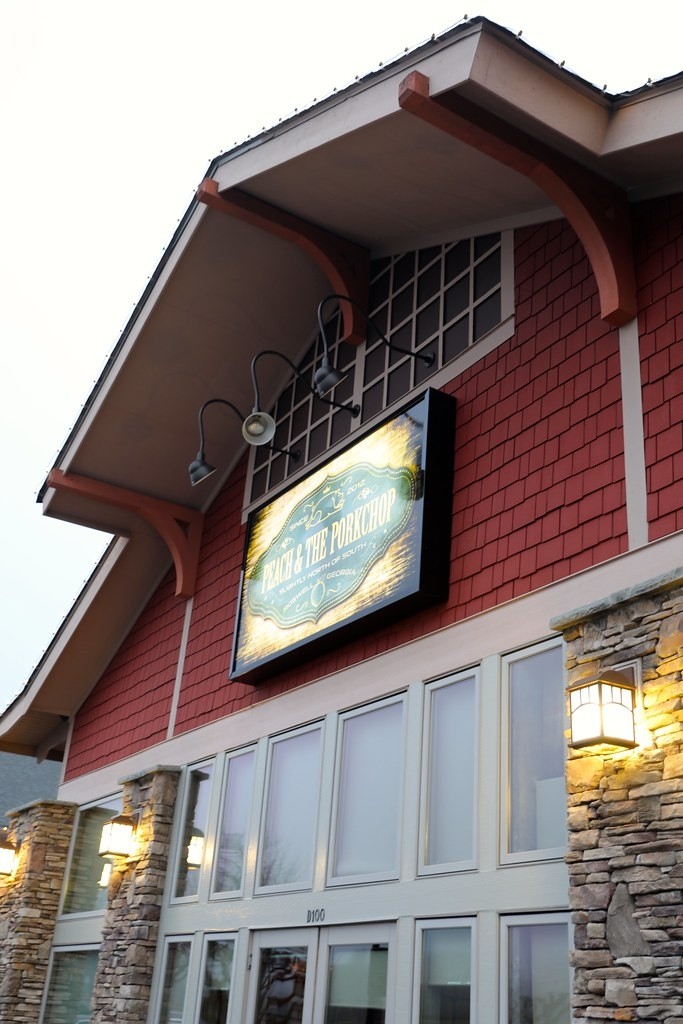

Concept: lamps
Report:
left=186, top=399, right=301, bottom=488
left=98, top=810, right=141, bottom=889
left=243, top=351, right=358, bottom=446
left=0, top=837, right=22, bottom=876
left=313, top=294, right=434, bottom=399
left=566, top=658, right=641, bottom=756
